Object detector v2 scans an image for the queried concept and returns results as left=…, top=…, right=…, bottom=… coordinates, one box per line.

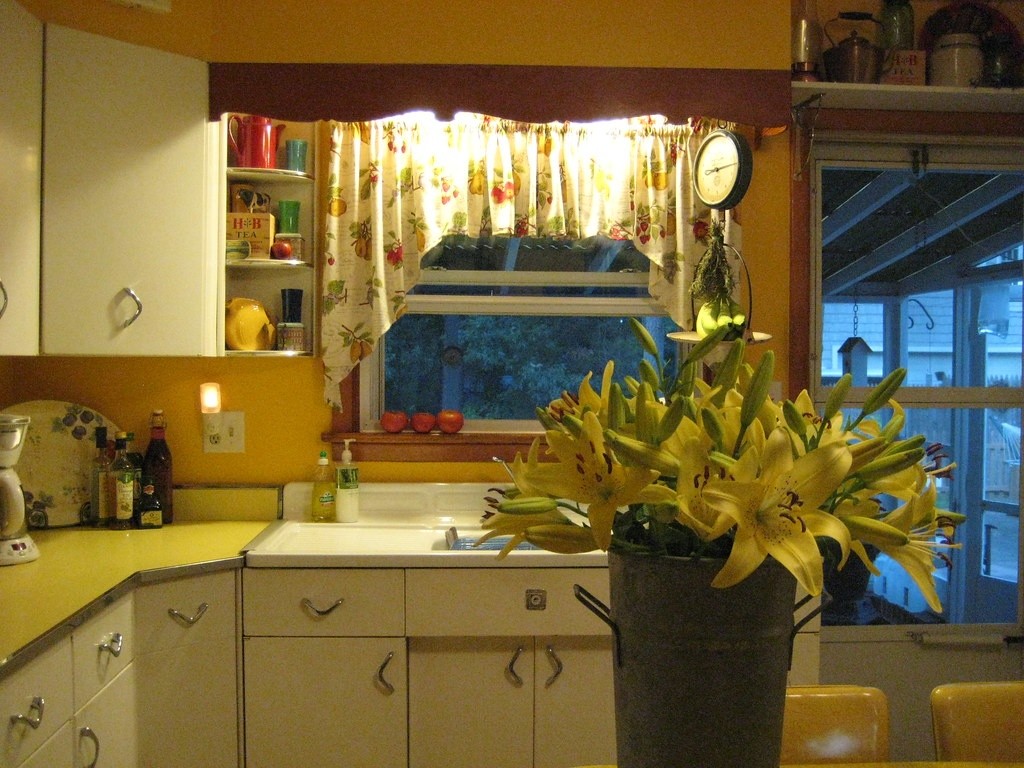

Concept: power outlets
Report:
left=202, top=411, right=245, bottom=454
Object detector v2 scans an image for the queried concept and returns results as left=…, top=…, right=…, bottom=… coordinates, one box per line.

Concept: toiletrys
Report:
left=312, top=450, right=335, bottom=522
left=333, top=438, right=362, bottom=524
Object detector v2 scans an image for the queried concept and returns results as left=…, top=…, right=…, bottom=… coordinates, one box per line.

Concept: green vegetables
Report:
left=689, top=219, right=737, bottom=305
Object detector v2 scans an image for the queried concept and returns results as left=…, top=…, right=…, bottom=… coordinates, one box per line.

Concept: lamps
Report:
left=199, top=381, right=222, bottom=413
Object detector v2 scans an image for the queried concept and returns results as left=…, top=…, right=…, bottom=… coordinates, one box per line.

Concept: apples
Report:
left=381, top=410, right=407, bottom=433
left=411, top=412, right=435, bottom=433
left=272, top=241, right=291, bottom=259
left=437, top=409, right=464, bottom=433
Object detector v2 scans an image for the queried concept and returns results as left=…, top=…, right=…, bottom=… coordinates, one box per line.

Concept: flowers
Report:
left=471, top=317, right=969, bottom=613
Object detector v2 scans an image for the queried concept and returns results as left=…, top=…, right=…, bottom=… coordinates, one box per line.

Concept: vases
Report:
left=574, top=535, right=834, bottom=768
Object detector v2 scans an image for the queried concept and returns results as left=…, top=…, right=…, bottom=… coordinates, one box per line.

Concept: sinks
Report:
left=428, top=531, right=546, bottom=553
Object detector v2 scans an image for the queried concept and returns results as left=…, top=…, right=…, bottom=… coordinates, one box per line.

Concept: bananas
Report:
left=695, top=293, right=746, bottom=341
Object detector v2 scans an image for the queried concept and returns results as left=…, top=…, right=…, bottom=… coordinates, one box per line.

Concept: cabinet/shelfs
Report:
left=216, top=109, right=323, bottom=358
left=0, top=0, right=209, bottom=358
left=0, top=566, right=821, bottom=768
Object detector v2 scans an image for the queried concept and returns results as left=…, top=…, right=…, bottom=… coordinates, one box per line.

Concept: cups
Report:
left=276, top=233, right=302, bottom=261
left=287, top=139, right=307, bottom=171
left=277, top=200, right=300, bottom=234
left=277, top=322, right=303, bottom=350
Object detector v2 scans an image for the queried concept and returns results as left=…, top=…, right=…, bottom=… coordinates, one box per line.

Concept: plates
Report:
left=1, top=399, right=120, bottom=526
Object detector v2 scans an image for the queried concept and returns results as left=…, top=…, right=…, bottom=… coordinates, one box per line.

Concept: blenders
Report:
left=0, top=413, right=41, bottom=567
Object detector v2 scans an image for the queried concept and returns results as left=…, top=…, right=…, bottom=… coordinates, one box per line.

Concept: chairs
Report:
left=930, top=680, right=1024, bottom=764
left=779, top=680, right=893, bottom=767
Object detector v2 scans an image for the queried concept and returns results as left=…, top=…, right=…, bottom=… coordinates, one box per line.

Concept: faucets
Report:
left=492, top=455, right=520, bottom=493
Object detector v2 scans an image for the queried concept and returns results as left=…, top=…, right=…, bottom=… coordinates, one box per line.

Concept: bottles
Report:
left=108, top=430, right=134, bottom=530
left=138, top=474, right=163, bottom=527
left=124, top=431, right=142, bottom=529
left=88, top=426, right=117, bottom=529
left=144, top=409, right=174, bottom=524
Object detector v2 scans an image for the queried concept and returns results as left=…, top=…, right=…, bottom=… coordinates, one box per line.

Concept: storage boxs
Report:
left=873, top=45, right=927, bottom=85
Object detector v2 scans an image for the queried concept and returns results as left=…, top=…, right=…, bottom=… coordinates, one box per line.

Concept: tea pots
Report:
left=228, top=113, right=286, bottom=168
